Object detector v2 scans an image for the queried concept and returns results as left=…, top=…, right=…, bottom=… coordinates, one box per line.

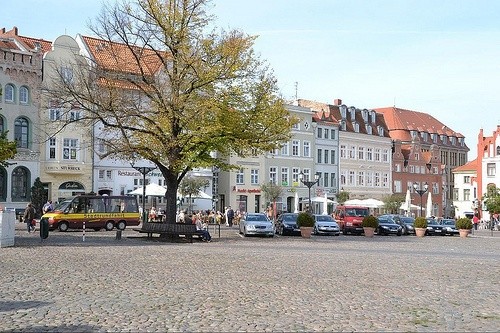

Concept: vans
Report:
left=334, top=206, right=371, bottom=236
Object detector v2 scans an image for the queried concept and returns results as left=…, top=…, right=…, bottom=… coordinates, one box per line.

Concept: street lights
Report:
left=298, top=170, right=321, bottom=216
left=130, top=160, right=159, bottom=233
left=413, top=179, right=429, bottom=218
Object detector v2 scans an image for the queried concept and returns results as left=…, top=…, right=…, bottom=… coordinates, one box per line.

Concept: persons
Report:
left=23, top=199, right=499, bottom=236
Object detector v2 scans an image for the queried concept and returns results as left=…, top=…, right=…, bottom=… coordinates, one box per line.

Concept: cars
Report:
left=439, top=219, right=461, bottom=236
left=275, top=212, right=304, bottom=236
left=399, top=217, right=417, bottom=235
left=423, top=218, right=447, bottom=236
left=239, top=212, right=275, bottom=238
left=311, top=214, right=340, bottom=237
left=376, top=216, right=402, bottom=237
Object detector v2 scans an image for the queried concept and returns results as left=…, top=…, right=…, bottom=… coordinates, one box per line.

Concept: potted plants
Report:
left=456, top=217, right=474, bottom=238
left=362, top=215, right=378, bottom=237
left=414, top=217, right=428, bottom=236
left=297, top=213, right=313, bottom=238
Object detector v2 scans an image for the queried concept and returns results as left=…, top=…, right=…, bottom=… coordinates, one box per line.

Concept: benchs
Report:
left=139, top=222, right=204, bottom=243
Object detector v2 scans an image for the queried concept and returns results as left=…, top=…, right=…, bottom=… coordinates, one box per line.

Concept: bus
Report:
left=41, top=195, right=141, bottom=231
